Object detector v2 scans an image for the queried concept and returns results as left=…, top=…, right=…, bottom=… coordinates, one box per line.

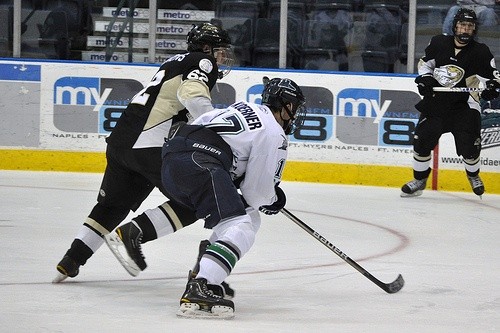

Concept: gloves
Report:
left=415, top=73, right=442, bottom=96
left=259, top=187, right=286, bottom=215
left=481, top=80, right=499, bottom=101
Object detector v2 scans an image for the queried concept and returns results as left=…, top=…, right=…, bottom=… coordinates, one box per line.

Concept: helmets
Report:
left=452, top=8, right=479, bottom=42
left=186, top=23, right=232, bottom=79
left=261, top=78, right=307, bottom=135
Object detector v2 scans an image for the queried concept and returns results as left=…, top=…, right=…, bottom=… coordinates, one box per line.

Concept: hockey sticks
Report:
left=279, top=204, right=405, bottom=292
left=428, top=85, right=499, bottom=92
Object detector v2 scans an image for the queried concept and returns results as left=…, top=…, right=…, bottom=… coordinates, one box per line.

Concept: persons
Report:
left=444, top=0, right=500, bottom=34
left=400, top=8, right=500, bottom=200
left=53, top=24, right=236, bottom=283
left=162, top=78, right=306, bottom=320
left=287, top=0, right=408, bottom=73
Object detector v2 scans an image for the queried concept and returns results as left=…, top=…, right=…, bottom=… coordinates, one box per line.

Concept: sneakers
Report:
left=467, top=174, right=484, bottom=200
left=400, top=177, right=427, bottom=197
left=186, top=240, right=235, bottom=300
left=52, top=255, right=79, bottom=284
left=176, top=278, right=236, bottom=319
left=104, top=221, right=147, bottom=277
